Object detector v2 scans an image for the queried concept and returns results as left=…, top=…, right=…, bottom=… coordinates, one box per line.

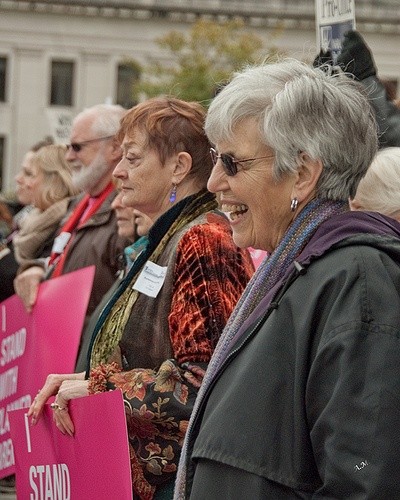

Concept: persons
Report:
left=0, top=28, right=400, bottom=500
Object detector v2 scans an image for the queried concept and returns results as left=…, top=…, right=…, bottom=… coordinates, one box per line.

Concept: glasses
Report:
left=209, top=148, right=303, bottom=176
left=66, top=135, right=113, bottom=152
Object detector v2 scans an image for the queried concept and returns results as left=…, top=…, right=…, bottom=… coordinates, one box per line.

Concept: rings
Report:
left=50, top=403, right=68, bottom=411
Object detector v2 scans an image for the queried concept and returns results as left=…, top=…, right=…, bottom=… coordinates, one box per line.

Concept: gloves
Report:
left=313, top=48, right=334, bottom=76
left=337, top=30, right=377, bottom=81
